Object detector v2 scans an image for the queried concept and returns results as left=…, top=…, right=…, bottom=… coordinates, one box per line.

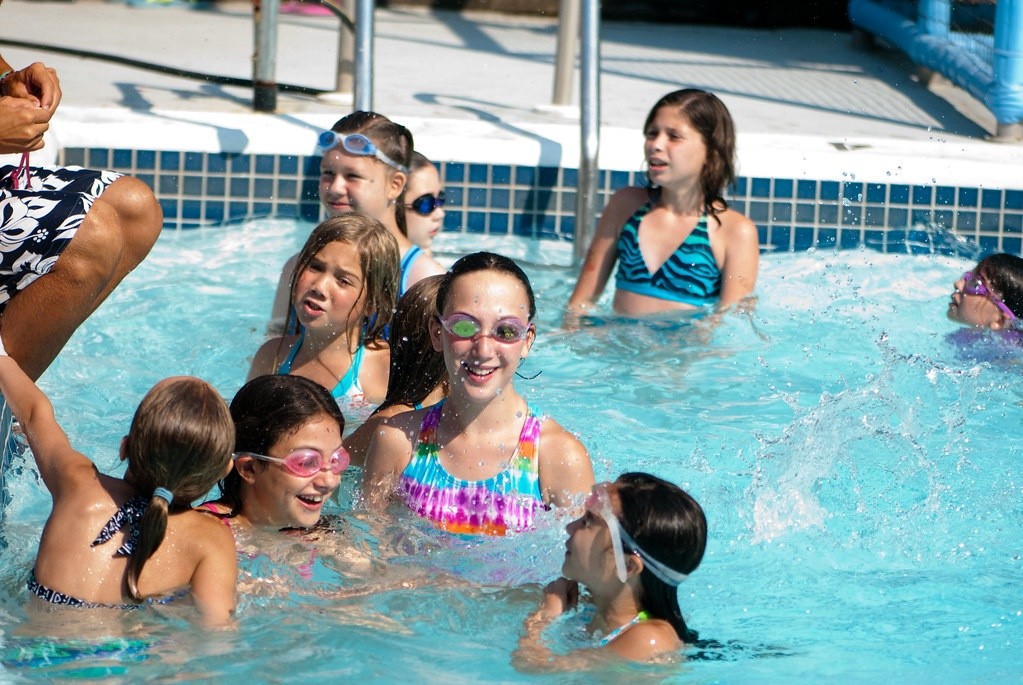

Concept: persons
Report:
left=564, top=87, right=760, bottom=343
left=3, top=340, right=245, bottom=685
left=366, top=249, right=596, bottom=589
left=242, top=212, right=446, bottom=443
left=401, top=149, right=448, bottom=251
left=192, top=375, right=492, bottom=638
left=509, top=471, right=708, bottom=685
left=261, top=110, right=448, bottom=342
left=946, top=253, right=1023, bottom=366
left=0, top=51, right=168, bottom=389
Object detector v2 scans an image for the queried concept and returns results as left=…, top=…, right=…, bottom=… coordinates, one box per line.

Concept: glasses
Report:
left=435, top=308, right=531, bottom=344
left=234, top=446, right=350, bottom=476
left=961, top=271, right=1015, bottom=321
left=318, top=131, right=409, bottom=173
left=582, top=481, right=689, bottom=588
left=405, top=191, right=446, bottom=215
